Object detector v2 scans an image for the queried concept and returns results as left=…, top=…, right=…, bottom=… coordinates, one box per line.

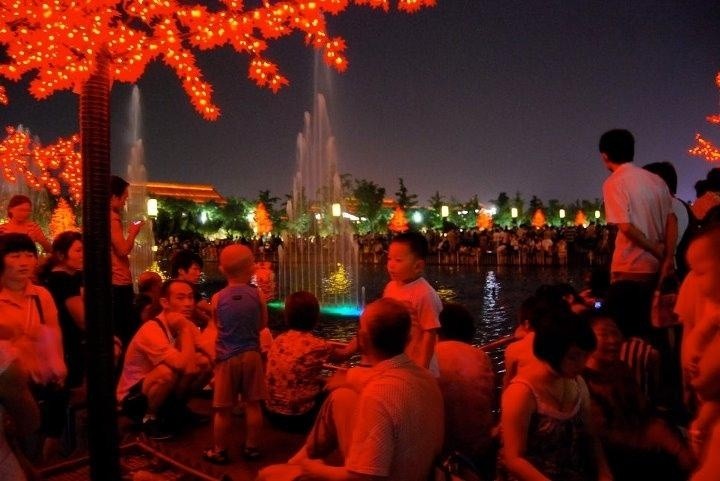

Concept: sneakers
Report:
left=141, top=418, right=173, bottom=440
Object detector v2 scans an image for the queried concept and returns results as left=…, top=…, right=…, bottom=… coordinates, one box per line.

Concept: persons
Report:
left=435, top=301, right=496, bottom=441
left=152, top=210, right=618, bottom=257
left=382, top=231, right=444, bottom=389
left=252, top=299, right=448, bottom=481
left=595, top=124, right=718, bottom=281
left=488, top=282, right=719, bottom=480
left=0, top=173, right=275, bottom=481
left=264, top=288, right=360, bottom=435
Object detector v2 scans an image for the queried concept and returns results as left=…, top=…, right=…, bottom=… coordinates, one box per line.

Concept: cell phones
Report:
left=134, top=219, right=142, bottom=225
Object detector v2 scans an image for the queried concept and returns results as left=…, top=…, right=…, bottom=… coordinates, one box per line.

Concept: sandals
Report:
left=241, top=444, right=263, bottom=462
left=202, top=445, right=230, bottom=464
